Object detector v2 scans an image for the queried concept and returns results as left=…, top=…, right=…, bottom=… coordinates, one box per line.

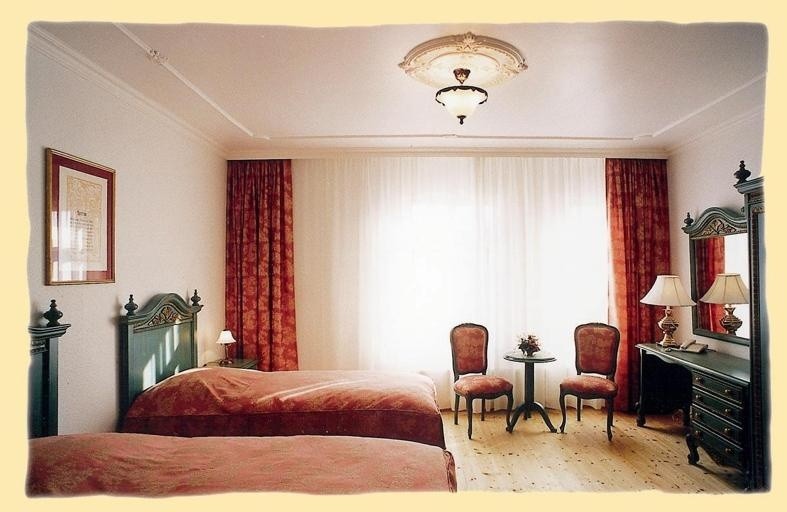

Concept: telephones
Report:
left=680, top=339, right=709, bottom=354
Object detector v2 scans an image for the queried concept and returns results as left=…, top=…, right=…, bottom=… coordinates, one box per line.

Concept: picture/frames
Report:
left=44, top=147, right=116, bottom=287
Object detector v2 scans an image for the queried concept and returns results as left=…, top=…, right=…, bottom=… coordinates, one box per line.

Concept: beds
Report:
left=118, top=290, right=446, bottom=448
left=25, top=298, right=457, bottom=499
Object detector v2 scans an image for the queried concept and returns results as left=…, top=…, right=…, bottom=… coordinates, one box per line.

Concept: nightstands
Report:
left=204, top=358, right=259, bottom=370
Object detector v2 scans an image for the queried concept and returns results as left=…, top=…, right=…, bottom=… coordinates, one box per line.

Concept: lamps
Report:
left=398, top=32, right=528, bottom=124
left=640, top=275, right=697, bottom=348
left=216, top=329, right=237, bottom=365
left=699, top=273, right=750, bottom=335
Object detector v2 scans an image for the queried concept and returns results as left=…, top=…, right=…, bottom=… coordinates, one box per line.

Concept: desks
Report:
left=503, top=350, right=557, bottom=434
left=635, top=342, right=750, bottom=489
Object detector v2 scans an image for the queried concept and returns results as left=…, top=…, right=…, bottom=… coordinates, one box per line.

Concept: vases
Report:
left=525, top=348, right=535, bottom=356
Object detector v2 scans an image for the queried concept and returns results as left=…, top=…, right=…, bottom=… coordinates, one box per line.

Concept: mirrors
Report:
left=681, top=206, right=750, bottom=346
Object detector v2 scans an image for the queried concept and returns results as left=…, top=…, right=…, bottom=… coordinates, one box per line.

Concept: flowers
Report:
left=518, top=335, right=540, bottom=354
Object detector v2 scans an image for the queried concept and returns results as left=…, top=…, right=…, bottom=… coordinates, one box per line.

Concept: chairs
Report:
left=559, top=322, right=620, bottom=441
left=450, top=323, right=514, bottom=439
